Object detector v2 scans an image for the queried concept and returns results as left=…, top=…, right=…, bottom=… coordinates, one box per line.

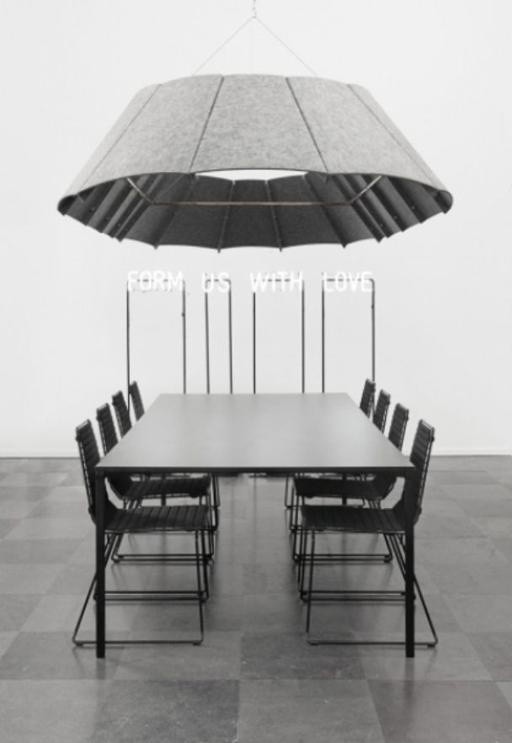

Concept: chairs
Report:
left=283, top=382, right=438, bottom=649
left=71, top=378, right=222, bottom=650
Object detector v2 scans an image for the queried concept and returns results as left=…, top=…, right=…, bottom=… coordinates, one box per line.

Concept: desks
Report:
left=93, top=388, right=415, bottom=661
